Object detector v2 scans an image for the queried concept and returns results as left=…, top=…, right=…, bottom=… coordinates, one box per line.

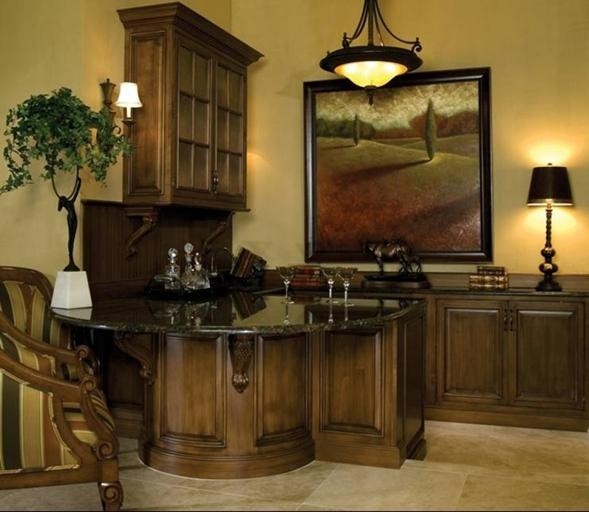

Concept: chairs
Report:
left=0, top=267, right=123, bottom=511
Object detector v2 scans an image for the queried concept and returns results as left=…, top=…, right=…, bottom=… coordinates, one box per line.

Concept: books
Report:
left=469, top=265, right=509, bottom=291
left=289, top=265, right=326, bottom=288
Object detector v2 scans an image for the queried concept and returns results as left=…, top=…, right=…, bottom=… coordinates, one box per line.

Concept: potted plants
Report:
left=0, top=86, right=134, bottom=308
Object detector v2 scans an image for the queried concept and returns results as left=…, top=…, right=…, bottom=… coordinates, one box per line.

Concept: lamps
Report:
left=319, top=1, right=423, bottom=105
left=525, top=163, right=575, bottom=291
left=95, top=77, right=143, bottom=153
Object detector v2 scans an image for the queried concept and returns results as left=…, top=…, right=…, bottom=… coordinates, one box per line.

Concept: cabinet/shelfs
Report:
left=117, top=2, right=266, bottom=212
left=425, top=293, right=588, bottom=434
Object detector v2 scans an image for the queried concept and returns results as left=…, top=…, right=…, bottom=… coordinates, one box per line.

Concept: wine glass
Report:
left=277, top=265, right=296, bottom=303
left=320, top=265, right=358, bottom=302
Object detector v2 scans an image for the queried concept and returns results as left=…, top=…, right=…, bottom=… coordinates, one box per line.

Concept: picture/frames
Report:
left=304, top=67, right=494, bottom=262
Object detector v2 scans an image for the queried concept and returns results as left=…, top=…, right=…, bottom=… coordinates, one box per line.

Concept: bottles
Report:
left=164, top=242, right=210, bottom=291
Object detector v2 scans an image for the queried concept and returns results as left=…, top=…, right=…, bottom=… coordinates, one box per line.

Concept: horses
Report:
left=358, top=236, right=424, bottom=275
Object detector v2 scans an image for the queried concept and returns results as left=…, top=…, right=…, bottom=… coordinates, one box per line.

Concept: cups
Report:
left=468, top=266, right=510, bottom=291
